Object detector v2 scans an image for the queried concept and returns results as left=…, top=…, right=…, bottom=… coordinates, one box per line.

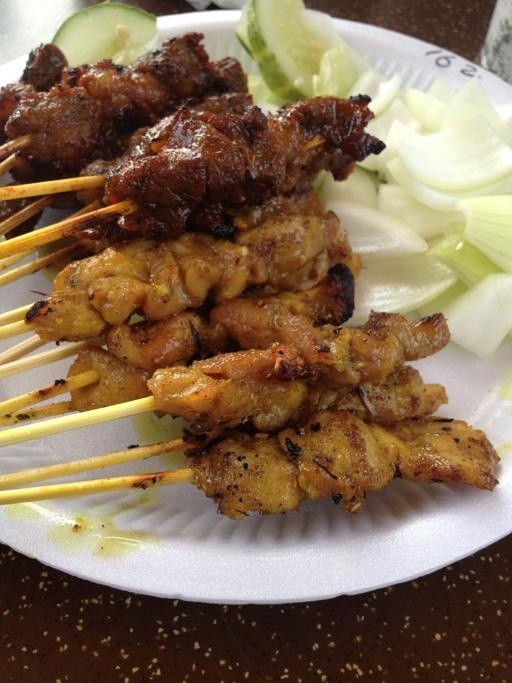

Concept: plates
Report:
left=1, top=11, right=511, bottom=607
